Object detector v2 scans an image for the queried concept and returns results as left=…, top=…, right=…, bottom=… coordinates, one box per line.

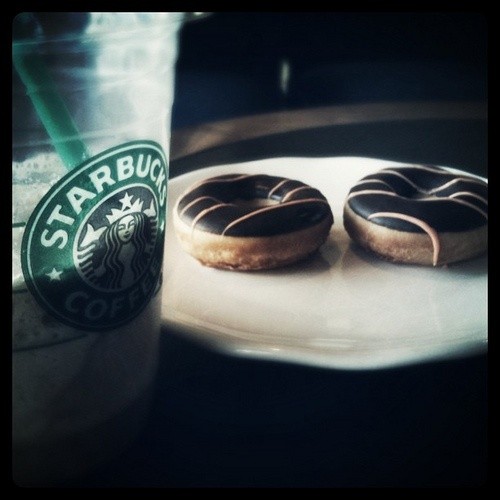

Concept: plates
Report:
left=159, top=156, right=488, bottom=369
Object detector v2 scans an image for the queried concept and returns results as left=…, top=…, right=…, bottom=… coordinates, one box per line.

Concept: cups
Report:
left=8, top=10, right=182, bottom=483
